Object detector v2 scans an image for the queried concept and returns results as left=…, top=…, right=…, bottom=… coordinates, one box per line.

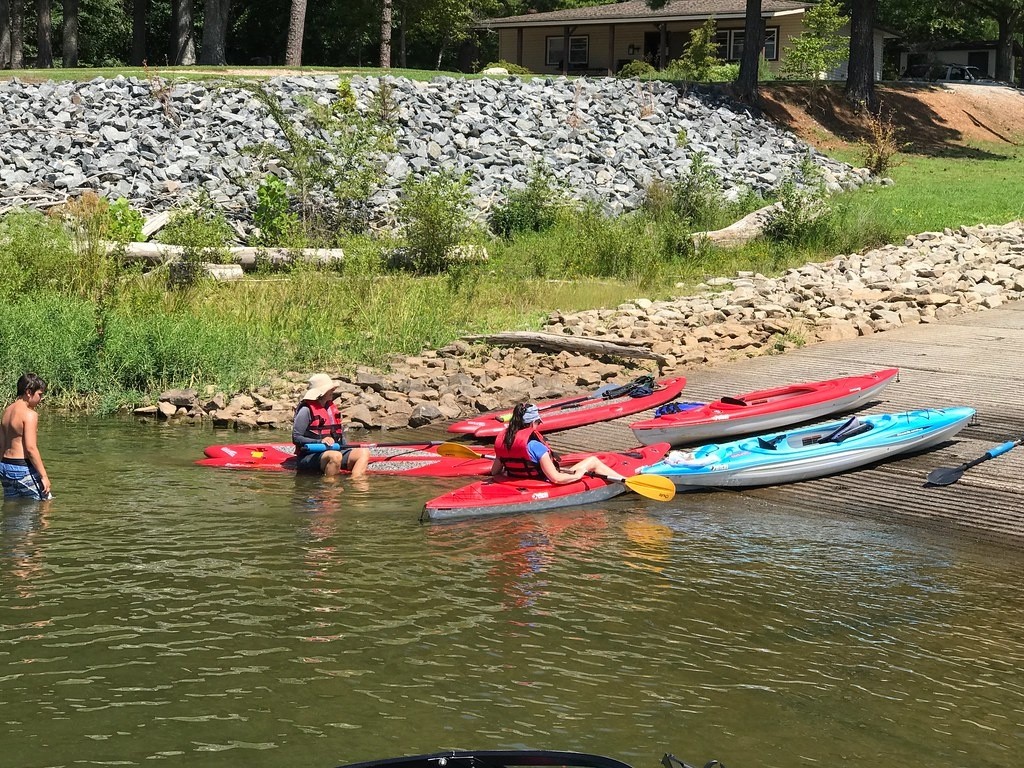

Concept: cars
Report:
left=5, top=57, right=54, bottom=68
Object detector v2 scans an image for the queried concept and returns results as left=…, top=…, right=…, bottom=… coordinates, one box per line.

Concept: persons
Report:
left=0, top=373, right=52, bottom=500
left=492, top=403, right=629, bottom=484
left=292, top=374, right=370, bottom=477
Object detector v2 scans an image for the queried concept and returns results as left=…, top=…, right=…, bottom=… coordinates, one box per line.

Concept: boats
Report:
left=629, top=369, right=900, bottom=447
left=425, top=442, right=671, bottom=518
left=640, top=406, right=977, bottom=486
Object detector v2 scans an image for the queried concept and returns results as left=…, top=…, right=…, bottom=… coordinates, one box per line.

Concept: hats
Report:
left=302, top=374, right=340, bottom=401
left=522, top=404, right=543, bottom=424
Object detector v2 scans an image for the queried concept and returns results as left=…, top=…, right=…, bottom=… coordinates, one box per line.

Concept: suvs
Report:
left=898, top=63, right=1018, bottom=88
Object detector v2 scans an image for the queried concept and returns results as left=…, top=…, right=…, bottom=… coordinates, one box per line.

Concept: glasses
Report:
left=537, top=419, right=541, bottom=425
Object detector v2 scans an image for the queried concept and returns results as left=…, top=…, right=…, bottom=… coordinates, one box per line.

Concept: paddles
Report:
left=436, top=442, right=677, bottom=503
left=926, top=437, right=1024, bottom=486
left=301, top=440, right=444, bottom=452
left=493, top=382, right=626, bottom=424
